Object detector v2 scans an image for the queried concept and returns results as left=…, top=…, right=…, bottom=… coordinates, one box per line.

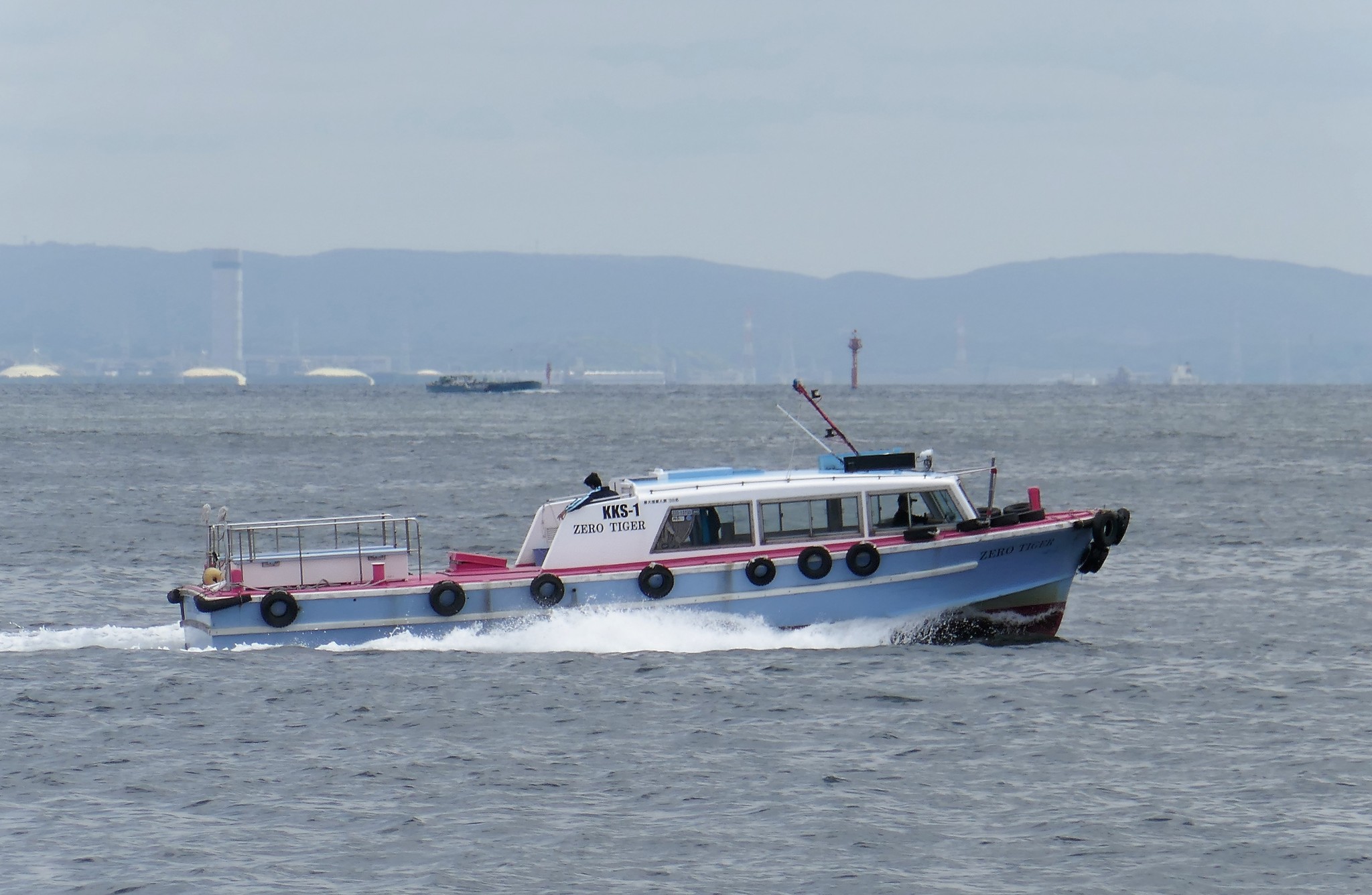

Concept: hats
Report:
left=898, top=494, right=918, bottom=504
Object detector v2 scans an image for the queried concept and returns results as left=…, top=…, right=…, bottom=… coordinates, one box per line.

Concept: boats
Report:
left=425, top=375, right=542, bottom=393
left=166, top=376, right=1133, bottom=654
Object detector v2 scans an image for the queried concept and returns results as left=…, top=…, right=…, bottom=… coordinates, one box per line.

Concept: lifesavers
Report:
left=1017, top=507, right=1045, bottom=523
left=1111, top=508, right=1131, bottom=546
left=797, top=545, right=833, bottom=580
left=260, top=590, right=299, bottom=628
left=976, top=507, right=1001, bottom=518
left=846, top=543, right=881, bottom=577
left=903, top=526, right=937, bottom=541
left=637, top=564, right=675, bottom=599
left=429, top=580, right=466, bottom=617
left=530, top=572, right=565, bottom=607
left=1078, top=541, right=1110, bottom=574
left=745, top=557, right=777, bottom=586
left=1002, top=502, right=1029, bottom=514
left=1092, top=509, right=1119, bottom=546
left=989, top=512, right=1019, bottom=528
left=956, top=517, right=991, bottom=532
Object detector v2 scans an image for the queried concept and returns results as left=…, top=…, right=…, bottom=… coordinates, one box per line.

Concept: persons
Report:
left=893, top=494, right=928, bottom=525
left=557, top=472, right=621, bottom=521
